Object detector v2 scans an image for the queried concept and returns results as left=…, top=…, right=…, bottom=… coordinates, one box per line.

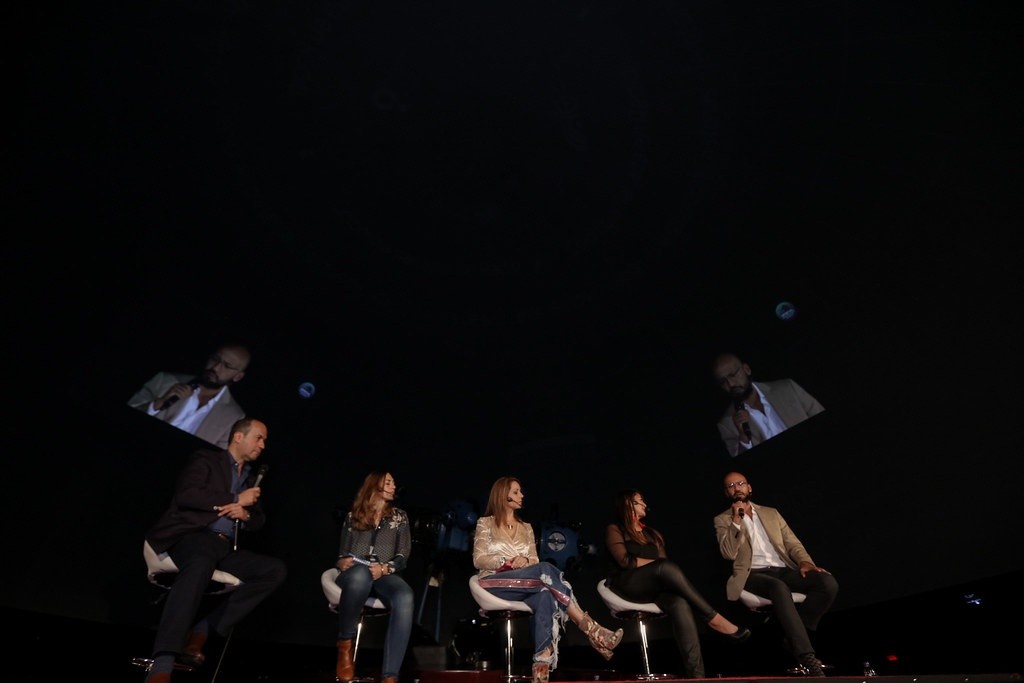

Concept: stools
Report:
left=469, top=575, right=534, bottom=683
left=321, top=567, right=386, bottom=683
left=131, top=539, right=243, bottom=674
left=597, top=578, right=674, bottom=681
left=739, top=590, right=830, bottom=676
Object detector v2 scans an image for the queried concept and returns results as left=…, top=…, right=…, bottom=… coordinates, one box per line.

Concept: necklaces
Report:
left=507, top=520, right=513, bottom=530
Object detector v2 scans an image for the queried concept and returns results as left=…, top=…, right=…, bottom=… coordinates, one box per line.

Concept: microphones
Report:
left=735, top=497, right=744, bottom=520
left=734, top=400, right=752, bottom=441
left=253, top=463, right=270, bottom=488
left=160, top=384, right=202, bottom=411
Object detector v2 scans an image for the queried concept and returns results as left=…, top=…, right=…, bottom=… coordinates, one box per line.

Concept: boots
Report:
left=382, top=676, right=397, bottom=683
left=577, top=611, right=623, bottom=660
left=530, top=662, right=550, bottom=683
left=336, top=638, right=356, bottom=682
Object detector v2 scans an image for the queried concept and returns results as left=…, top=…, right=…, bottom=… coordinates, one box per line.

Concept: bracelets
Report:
left=381, top=561, right=395, bottom=577
left=502, top=557, right=506, bottom=565
left=524, top=557, right=530, bottom=564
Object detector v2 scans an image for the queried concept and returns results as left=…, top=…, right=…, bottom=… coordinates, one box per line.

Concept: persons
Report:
left=473, top=478, right=623, bottom=683
left=143, top=418, right=270, bottom=683
left=604, top=488, right=750, bottom=679
left=713, top=472, right=838, bottom=677
left=127, top=344, right=251, bottom=450
left=712, top=353, right=825, bottom=457
left=336, top=471, right=414, bottom=683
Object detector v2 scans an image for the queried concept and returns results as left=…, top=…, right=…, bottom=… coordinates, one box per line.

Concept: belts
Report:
left=197, top=527, right=235, bottom=545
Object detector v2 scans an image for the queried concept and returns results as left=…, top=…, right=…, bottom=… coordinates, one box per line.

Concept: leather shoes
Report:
left=145, top=670, right=172, bottom=683
left=182, top=630, right=210, bottom=666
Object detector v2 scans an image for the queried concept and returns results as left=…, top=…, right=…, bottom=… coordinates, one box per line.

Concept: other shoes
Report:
left=797, top=653, right=826, bottom=677
left=860, top=659, right=878, bottom=677
left=702, top=612, right=751, bottom=642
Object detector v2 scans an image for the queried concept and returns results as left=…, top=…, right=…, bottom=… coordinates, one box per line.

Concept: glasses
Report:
left=719, top=365, right=742, bottom=388
left=207, top=353, right=240, bottom=372
left=724, top=480, right=748, bottom=491
